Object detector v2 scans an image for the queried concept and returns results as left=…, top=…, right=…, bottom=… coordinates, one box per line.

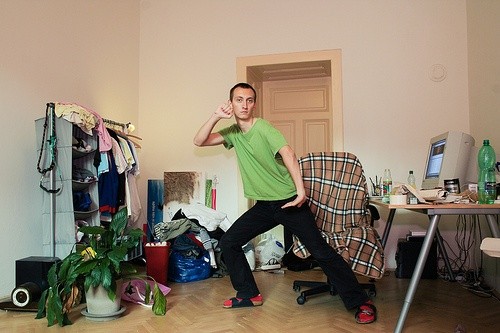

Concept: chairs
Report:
left=283, top=152, right=382, bottom=305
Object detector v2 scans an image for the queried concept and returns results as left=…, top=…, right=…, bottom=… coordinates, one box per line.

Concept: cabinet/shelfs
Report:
left=35, top=114, right=100, bottom=262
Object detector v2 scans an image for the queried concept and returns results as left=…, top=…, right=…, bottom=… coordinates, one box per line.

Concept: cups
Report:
left=371, top=185, right=383, bottom=196
left=389, top=195, right=407, bottom=205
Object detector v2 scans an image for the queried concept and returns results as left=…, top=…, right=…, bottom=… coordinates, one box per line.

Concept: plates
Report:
left=367, top=196, right=383, bottom=200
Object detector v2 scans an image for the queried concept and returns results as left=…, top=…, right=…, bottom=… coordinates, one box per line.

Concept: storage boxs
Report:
left=143, top=241, right=171, bottom=286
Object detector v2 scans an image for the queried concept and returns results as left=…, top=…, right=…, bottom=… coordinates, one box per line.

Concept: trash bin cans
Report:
left=144, top=242, right=171, bottom=286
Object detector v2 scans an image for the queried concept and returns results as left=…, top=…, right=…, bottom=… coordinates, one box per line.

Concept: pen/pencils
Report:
left=376, top=175, right=377, bottom=185
left=379, top=177, right=382, bottom=184
left=369, top=177, right=375, bottom=186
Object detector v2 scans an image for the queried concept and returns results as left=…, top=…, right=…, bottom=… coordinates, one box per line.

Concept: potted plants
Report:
left=35, top=207, right=167, bottom=327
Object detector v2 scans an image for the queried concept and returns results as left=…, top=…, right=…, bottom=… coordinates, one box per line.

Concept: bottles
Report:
left=445, top=181, right=458, bottom=196
left=382, top=169, right=392, bottom=202
left=407, top=171, right=415, bottom=203
left=478, top=140, right=496, bottom=204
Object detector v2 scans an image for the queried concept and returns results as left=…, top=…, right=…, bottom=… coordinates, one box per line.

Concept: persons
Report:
left=193, top=82, right=378, bottom=324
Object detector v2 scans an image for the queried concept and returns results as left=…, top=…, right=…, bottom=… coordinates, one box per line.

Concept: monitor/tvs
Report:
left=418, top=130, right=475, bottom=200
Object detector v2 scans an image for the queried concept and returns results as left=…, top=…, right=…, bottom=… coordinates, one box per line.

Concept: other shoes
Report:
left=355, top=302, right=377, bottom=323
left=223, top=293, right=263, bottom=307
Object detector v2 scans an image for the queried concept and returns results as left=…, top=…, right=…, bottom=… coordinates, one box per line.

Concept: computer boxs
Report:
left=395, top=238, right=437, bottom=280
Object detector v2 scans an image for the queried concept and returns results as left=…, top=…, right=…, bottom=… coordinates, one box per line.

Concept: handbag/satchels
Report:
left=170, top=251, right=212, bottom=283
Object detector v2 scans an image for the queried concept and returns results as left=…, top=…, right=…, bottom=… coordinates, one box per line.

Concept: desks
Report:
left=368, top=196, right=500, bottom=333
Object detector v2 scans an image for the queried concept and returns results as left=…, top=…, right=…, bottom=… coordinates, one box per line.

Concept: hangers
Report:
left=101, top=118, right=142, bottom=149
